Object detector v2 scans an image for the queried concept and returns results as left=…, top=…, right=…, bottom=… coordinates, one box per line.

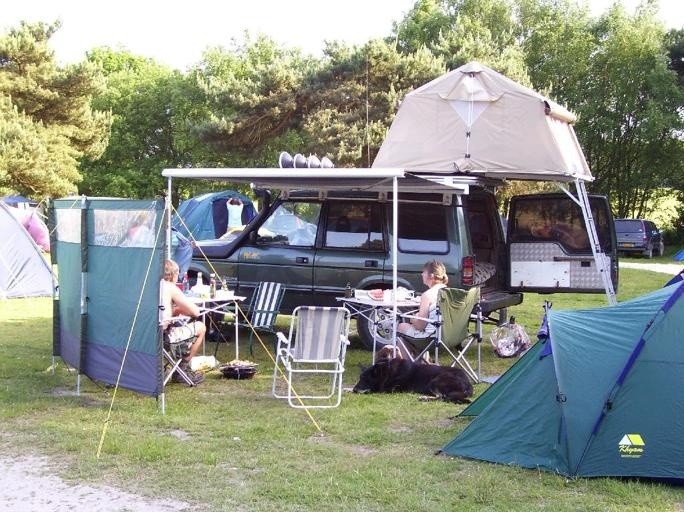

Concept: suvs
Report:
left=614, top=221, right=664, bottom=258
left=168, top=170, right=619, bottom=352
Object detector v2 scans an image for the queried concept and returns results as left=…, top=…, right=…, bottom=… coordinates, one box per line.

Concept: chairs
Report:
left=395, top=287, right=481, bottom=384
left=159, top=305, right=200, bottom=389
left=212, top=281, right=288, bottom=357
left=272, top=306, right=350, bottom=408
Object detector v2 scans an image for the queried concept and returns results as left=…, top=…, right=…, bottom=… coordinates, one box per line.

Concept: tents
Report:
left=176, top=190, right=258, bottom=245
left=672, top=248, right=684, bottom=262
left=12, top=206, right=51, bottom=252
left=0, top=198, right=59, bottom=299
left=437, top=267, right=684, bottom=481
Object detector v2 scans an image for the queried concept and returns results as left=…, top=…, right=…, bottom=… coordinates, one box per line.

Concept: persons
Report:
left=398, top=260, right=448, bottom=363
left=159, top=260, right=208, bottom=383
left=225, top=197, right=244, bottom=231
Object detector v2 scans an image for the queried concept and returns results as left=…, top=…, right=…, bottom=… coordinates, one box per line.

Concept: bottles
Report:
left=182, top=272, right=189, bottom=291
left=197, top=272, right=202, bottom=289
left=210, top=272, right=216, bottom=300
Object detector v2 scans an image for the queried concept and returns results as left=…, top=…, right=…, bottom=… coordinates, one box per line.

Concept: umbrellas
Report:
left=371, top=60, right=621, bottom=307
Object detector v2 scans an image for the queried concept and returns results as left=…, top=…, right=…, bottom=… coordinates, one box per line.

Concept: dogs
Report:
left=354, top=359, right=473, bottom=404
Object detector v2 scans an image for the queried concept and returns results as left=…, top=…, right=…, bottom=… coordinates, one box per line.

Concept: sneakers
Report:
left=173, top=368, right=205, bottom=385
